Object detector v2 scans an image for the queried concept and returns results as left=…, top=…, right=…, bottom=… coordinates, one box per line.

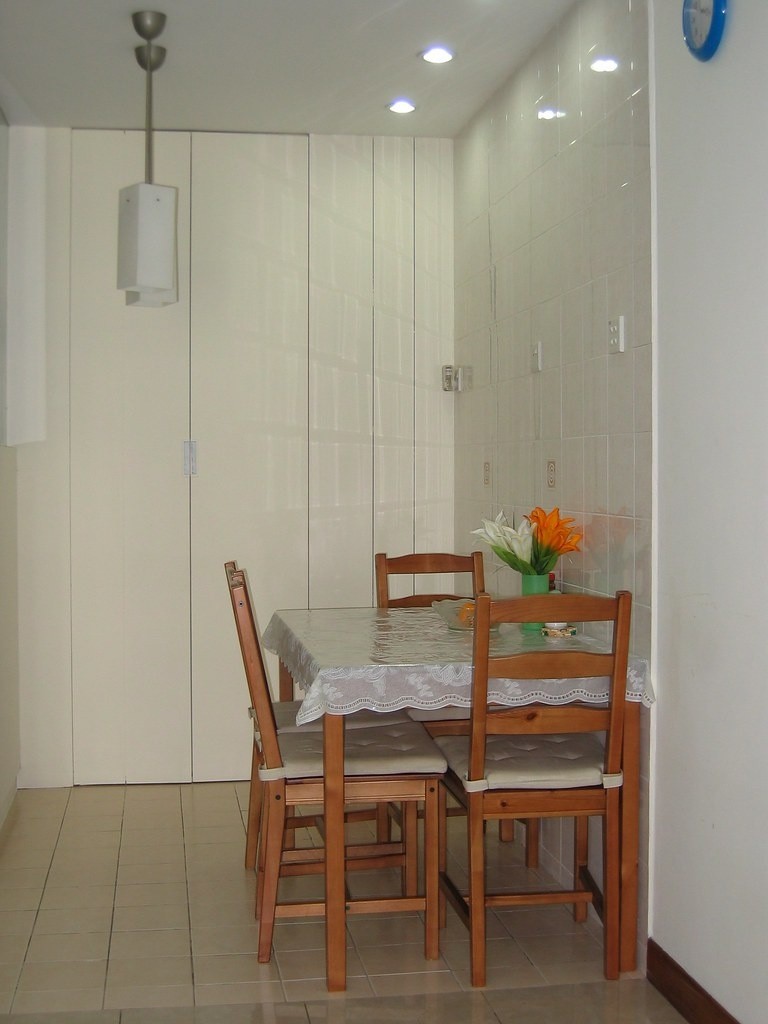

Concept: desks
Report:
left=259, top=608, right=656, bottom=992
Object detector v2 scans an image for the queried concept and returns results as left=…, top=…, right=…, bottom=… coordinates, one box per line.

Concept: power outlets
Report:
left=546, top=458, right=558, bottom=489
left=482, top=460, right=490, bottom=485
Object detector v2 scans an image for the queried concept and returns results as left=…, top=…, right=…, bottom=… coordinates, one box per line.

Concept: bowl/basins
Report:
left=541, top=627, right=577, bottom=643
left=432, top=599, right=500, bottom=631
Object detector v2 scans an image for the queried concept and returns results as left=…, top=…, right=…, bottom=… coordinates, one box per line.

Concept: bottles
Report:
left=545, top=573, right=567, bottom=629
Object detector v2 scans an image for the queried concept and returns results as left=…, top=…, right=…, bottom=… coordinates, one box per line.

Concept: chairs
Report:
left=378, top=550, right=486, bottom=846
left=230, top=575, right=446, bottom=963
left=224, top=558, right=413, bottom=897
left=437, top=588, right=635, bottom=986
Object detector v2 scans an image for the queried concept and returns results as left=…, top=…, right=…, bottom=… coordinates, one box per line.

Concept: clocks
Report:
left=682, top=0, right=726, bottom=62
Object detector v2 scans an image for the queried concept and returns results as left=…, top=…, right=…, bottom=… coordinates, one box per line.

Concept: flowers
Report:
left=467, top=509, right=587, bottom=575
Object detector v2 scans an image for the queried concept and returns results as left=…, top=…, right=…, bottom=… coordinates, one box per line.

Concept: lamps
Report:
left=115, top=11, right=179, bottom=295
left=124, top=46, right=179, bottom=308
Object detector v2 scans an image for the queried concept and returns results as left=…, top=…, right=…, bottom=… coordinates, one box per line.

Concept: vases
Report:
left=521, top=573, right=550, bottom=633
